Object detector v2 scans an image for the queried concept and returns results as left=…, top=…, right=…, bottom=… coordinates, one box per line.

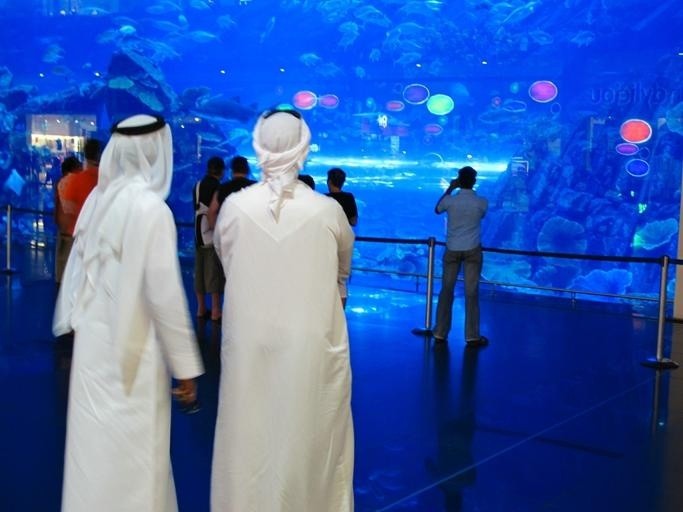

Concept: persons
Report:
left=209, top=108, right=356, bottom=512
left=192, top=157, right=224, bottom=322
left=207, top=155, right=258, bottom=231
left=53, top=138, right=103, bottom=296
left=50, top=113, right=206, bottom=511
left=324, top=168, right=357, bottom=227
left=432, top=166, right=489, bottom=347
left=297, top=174, right=316, bottom=192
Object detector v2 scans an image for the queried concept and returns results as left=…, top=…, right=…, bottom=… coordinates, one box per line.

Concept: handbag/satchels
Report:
left=195, top=202, right=218, bottom=248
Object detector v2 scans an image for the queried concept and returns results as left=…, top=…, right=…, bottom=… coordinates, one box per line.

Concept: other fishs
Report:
left=0, top=0, right=596, bottom=260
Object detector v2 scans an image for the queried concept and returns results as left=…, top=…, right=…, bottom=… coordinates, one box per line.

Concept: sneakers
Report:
left=436, top=336, right=488, bottom=345
